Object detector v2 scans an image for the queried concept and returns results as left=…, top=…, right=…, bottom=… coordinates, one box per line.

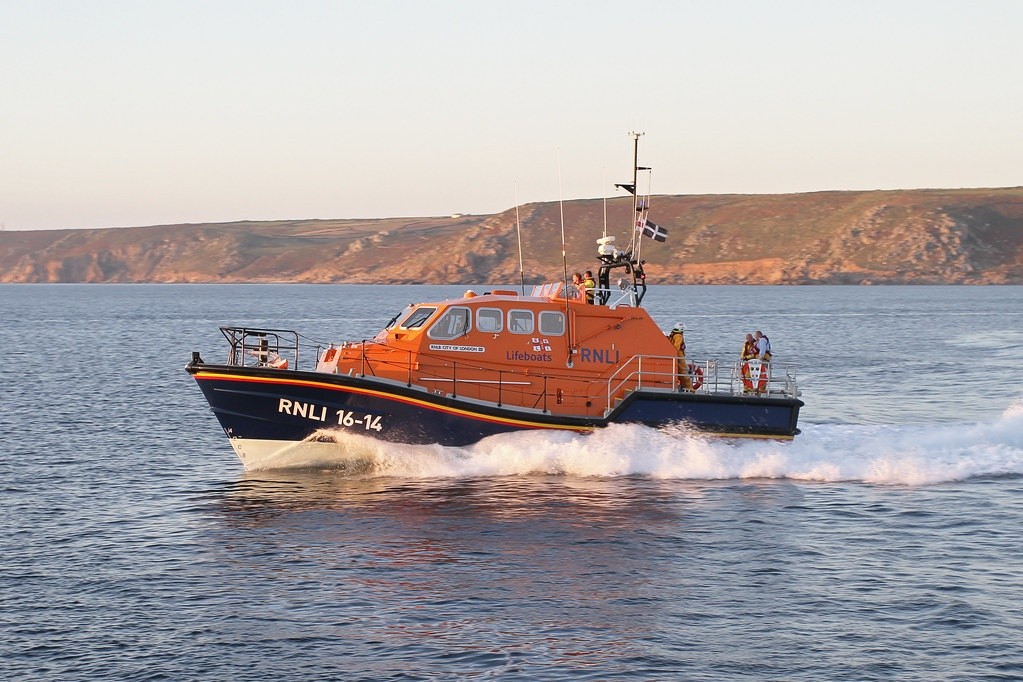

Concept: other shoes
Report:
left=759, top=390, right=766, bottom=393
left=684, top=388, right=696, bottom=391
left=743, top=387, right=749, bottom=393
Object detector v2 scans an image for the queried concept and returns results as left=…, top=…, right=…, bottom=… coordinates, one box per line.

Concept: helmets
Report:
left=673, top=322, right=687, bottom=332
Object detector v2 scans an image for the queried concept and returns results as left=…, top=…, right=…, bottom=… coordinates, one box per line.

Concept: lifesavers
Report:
left=688, top=366, right=704, bottom=390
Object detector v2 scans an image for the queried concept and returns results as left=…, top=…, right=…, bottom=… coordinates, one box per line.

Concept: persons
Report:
left=571, top=271, right=596, bottom=305
left=667, top=322, right=697, bottom=393
left=740, top=331, right=773, bottom=394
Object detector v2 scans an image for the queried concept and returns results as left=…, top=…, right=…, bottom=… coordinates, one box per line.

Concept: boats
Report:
left=185, top=130, right=806, bottom=473
